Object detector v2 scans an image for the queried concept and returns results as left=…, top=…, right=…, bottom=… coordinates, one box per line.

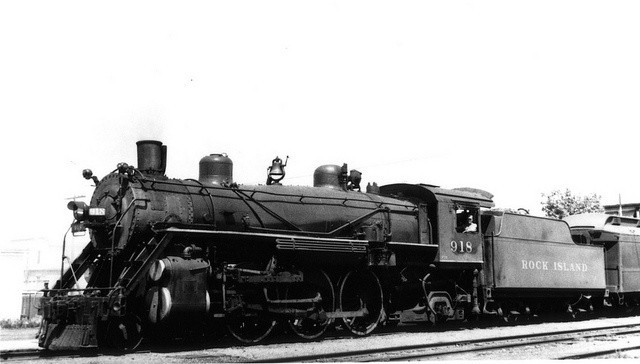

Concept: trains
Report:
left=34, top=140, right=637, bottom=356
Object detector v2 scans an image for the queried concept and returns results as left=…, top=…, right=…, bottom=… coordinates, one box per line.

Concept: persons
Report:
left=462, top=214, right=477, bottom=232
